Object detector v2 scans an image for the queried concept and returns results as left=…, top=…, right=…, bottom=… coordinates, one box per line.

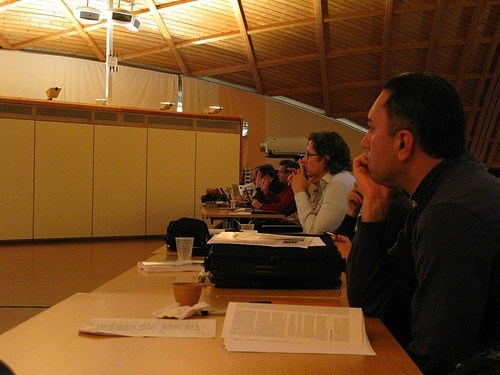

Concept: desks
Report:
left=0, top=244, right=426, bottom=375
left=204, top=208, right=286, bottom=229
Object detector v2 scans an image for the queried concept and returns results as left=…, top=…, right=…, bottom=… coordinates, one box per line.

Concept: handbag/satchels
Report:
left=202, top=233, right=343, bottom=289
left=165, top=217, right=210, bottom=257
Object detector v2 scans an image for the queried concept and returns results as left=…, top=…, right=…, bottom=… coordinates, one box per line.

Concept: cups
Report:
left=171, top=282, right=203, bottom=307
left=240, top=223, right=254, bottom=230
left=229, top=200, right=236, bottom=208
left=175, top=237, right=194, bottom=261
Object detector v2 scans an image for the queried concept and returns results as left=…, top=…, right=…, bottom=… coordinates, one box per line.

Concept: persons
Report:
left=206, top=131, right=363, bottom=260
left=346, top=71, right=500, bottom=375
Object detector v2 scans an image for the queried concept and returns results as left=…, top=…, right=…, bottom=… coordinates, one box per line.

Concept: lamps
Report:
left=75, top=0, right=142, bottom=32
left=206, top=106, right=224, bottom=114
left=46, top=88, right=60, bottom=99
left=160, top=102, right=174, bottom=111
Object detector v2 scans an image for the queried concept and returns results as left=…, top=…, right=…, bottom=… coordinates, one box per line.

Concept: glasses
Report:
left=306, top=151, right=324, bottom=159
